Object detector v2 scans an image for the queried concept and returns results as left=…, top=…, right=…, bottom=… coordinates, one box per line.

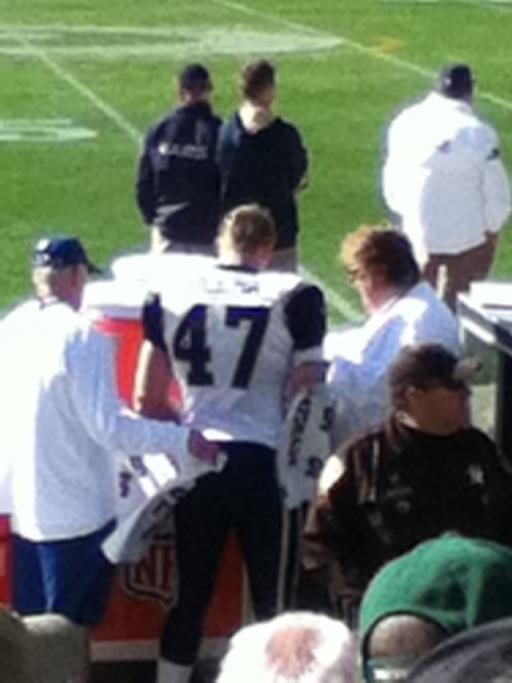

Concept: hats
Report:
left=439, top=63, right=470, bottom=91
left=387, top=342, right=482, bottom=397
left=357, top=531, right=511, bottom=682
left=30, top=234, right=105, bottom=276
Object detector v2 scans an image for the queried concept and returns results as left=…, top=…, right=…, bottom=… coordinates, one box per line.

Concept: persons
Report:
left=379, top=58, right=510, bottom=312
left=214, top=57, right=307, bottom=268
left=132, top=64, right=223, bottom=257
left=1, top=202, right=325, bottom=682
left=215, top=224, right=511, bottom=683
